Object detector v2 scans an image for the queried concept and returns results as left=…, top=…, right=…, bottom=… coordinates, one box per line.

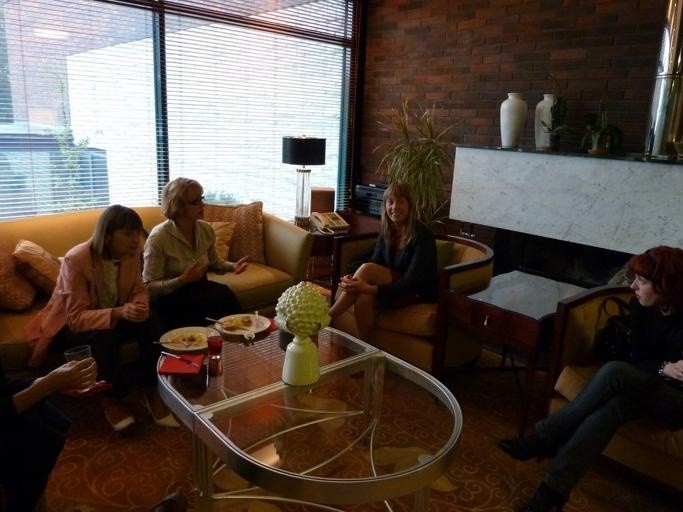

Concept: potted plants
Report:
left=541, top=79, right=631, bottom=156
left=274, top=281, right=333, bottom=386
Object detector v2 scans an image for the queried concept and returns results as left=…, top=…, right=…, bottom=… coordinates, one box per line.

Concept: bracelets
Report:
left=658, top=360, right=671, bottom=377
left=160, top=280, right=176, bottom=294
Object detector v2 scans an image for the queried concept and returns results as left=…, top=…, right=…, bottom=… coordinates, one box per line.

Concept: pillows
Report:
left=197, top=204, right=266, bottom=264
left=1, top=238, right=61, bottom=311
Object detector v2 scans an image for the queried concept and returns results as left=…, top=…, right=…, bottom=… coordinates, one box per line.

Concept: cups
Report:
left=63, top=345, right=97, bottom=394
left=207, top=324, right=223, bottom=359
left=206, top=354, right=223, bottom=392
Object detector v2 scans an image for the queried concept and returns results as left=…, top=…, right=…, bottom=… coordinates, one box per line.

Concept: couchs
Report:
left=1, top=204, right=313, bottom=383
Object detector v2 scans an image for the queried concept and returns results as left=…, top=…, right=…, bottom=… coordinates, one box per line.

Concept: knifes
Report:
left=161, top=351, right=201, bottom=368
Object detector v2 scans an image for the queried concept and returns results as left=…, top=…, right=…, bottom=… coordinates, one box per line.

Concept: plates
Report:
left=159, top=326, right=221, bottom=352
left=215, top=313, right=271, bottom=336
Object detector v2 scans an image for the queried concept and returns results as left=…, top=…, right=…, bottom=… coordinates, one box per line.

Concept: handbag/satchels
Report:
left=603, top=296, right=632, bottom=363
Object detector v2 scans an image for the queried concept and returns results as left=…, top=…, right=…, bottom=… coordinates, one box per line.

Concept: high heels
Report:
left=496, top=434, right=556, bottom=462
left=144, top=393, right=181, bottom=428
left=104, top=407, right=135, bottom=431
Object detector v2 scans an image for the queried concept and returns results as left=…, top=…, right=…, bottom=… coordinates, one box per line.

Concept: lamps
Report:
left=283, top=138, right=327, bottom=211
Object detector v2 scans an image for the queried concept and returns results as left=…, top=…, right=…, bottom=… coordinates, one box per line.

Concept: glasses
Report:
left=188, top=195, right=205, bottom=206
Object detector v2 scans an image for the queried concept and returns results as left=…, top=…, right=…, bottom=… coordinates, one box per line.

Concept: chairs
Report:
left=536, top=285, right=683, bottom=499
left=330, top=232, right=495, bottom=394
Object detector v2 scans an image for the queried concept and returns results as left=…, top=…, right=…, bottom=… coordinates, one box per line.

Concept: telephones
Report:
left=310, top=212, right=350, bottom=230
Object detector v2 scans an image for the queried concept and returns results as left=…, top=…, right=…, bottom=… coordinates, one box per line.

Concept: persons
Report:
left=328, top=180, right=439, bottom=342
left=23, top=204, right=181, bottom=440
left=495, top=246, right=683, bottom=512
left=142, top=176, right=250, bottom=329
left=0, top=356, right=113, bottom=512
